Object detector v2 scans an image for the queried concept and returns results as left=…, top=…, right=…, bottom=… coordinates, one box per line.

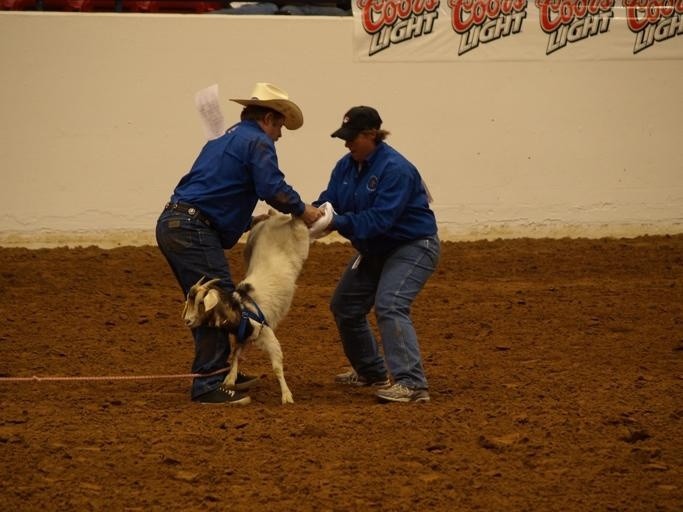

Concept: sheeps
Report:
left=181, top=201, right=338, bottom=405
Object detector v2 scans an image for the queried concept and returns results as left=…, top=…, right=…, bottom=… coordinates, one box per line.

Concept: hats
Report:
left=229, top=81, right=303, bottom=130
left=330, top=106, right=383, bottom=139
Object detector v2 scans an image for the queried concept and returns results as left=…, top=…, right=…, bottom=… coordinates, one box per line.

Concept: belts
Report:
left=165, top=201, right=217, bottom=229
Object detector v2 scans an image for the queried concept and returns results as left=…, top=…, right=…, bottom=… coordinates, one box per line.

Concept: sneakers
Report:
left=221, top=370, right=258, bottom=388
left=191, top=386, right=252, bottom=406
left=335, top=369, right=391, bottom=387
left=374, top=382, right=430, bottom=402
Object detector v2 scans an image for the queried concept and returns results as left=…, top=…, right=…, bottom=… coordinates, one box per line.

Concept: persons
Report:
left=155, top=82, right=324, bottom=406
left=311, top=105, right=441, bottom=403
